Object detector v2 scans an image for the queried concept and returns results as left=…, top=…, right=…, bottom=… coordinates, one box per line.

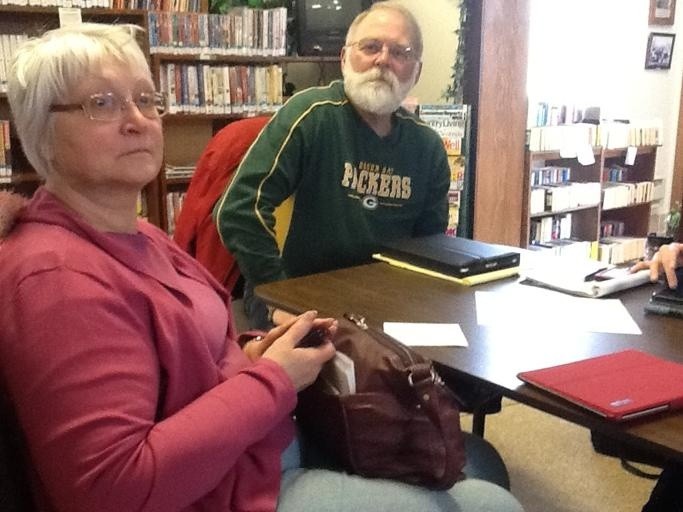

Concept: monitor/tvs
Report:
left=300, top=2, right=369, bottom=55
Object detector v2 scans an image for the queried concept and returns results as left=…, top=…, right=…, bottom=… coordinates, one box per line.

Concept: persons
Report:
left=0, top=24, right=523, bottom=512
left=216, top=2, right=451, bottom=330
left=630, top=243, right=683, bottom=289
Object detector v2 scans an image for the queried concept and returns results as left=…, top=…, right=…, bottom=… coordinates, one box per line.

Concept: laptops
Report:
left=516, top=349, right=683, bottom=423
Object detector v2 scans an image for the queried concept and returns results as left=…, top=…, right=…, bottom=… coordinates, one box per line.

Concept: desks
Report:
left=254, top=244, right=683, bottom=470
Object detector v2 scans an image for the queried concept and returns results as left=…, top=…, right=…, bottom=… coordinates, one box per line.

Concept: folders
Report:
left=379, top=232, right=520, bottom=279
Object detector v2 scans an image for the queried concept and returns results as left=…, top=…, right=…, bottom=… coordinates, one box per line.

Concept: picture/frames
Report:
left=649, top=0, right=676, bottom=25
left=645, top=32, right=675, bottom=69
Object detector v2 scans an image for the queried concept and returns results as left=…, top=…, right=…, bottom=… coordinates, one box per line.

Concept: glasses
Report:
left=49, top=90, right=170, bottom=121
left=344, top=37, right=416, bottom=63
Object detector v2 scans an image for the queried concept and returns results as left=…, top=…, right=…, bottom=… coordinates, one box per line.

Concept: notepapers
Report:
left=575, top=145, right=595, bottom=166
left=58, top=8, right=82, bottom=29
left=625, top=147, right=638, bottom=166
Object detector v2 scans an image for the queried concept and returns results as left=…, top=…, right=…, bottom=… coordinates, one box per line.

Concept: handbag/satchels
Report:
left=291, top=309, right=468, bottom=493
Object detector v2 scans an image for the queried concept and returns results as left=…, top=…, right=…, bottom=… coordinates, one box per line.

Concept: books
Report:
left=531, top=167, right=600, bottom=213
left=165, top=165, right=195, bottom=179
left=416, top=104, right=466, bottom=155
left=521, top=252, right=651, bottom=297
left=606, top=120, right=662, bottom=148
left=529, top=213, right=591, bottom=258
left=159, top=64, right=282, bottom=114
left=149, top=8, right=287, bottom=57
left=0, top=120, right=12, bottom=176
left=526, top=103, right=599, bottom=151
left=166, top=193, right=185, bottom=240
left=603, top=167, right=654, bottom=210
left=446, top=190, right=461, bottom=237
left=598, top=221, right=648, bottom=264
left=446, top=155, right=465, bottom=188
left=1, top=35, right=29, bottom=92
left=0, top=0, right=201, bottom=13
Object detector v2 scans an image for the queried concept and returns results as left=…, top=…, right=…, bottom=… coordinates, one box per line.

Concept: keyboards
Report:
left=644, top=275, right=682, bottom=318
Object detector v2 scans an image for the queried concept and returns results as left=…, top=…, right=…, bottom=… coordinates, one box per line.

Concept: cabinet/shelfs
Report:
left=519, top=149, right=657, bottom=248
left=1, top=1, right=292, bottom=237
